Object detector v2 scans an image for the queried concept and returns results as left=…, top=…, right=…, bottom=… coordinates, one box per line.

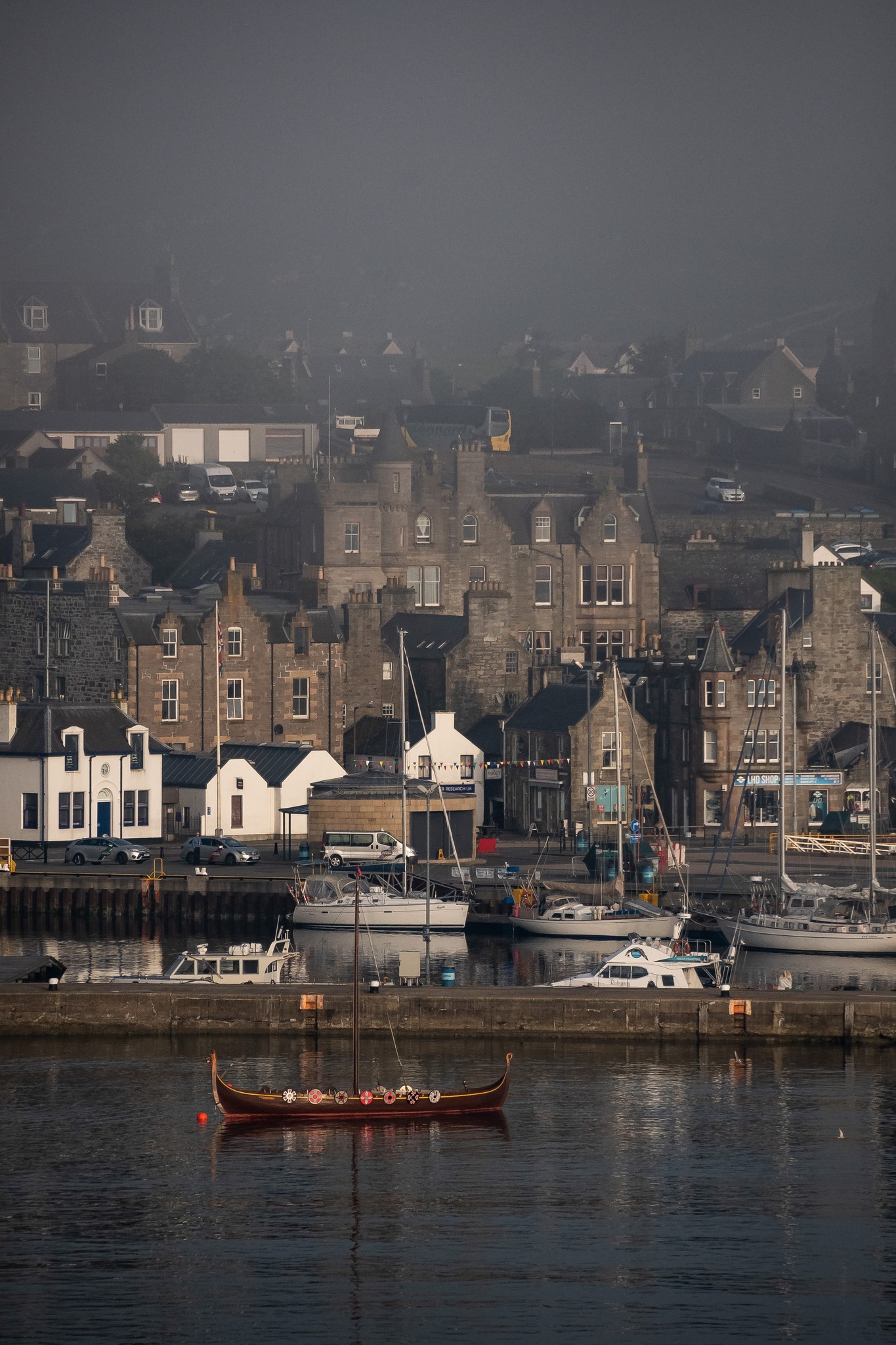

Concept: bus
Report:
left=395, top=403, right=513, bottom=452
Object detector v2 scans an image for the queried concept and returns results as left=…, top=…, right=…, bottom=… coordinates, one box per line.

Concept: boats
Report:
left=108, top=913, right=301, bottom=984
left=1, top=956, right=67, bottom=983
left=532, top=911, right=742, bottom=989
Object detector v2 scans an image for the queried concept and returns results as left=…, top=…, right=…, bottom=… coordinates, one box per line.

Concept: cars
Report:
left=65, top=834, right=151, bottom=865
left=704, top=478, right=748, bottom=504
left=179, top=834, right=261, bottom=865
left=146, top=460, right=269, bottom=506
left=835, top=544, right=896, bottom=569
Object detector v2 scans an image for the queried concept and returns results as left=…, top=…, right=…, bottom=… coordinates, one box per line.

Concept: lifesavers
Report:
left=524, top=893, right=537, bottom=908
left=673, top=940, right=690, bottom=956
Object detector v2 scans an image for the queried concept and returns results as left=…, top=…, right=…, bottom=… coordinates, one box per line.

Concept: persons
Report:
left=192, top=832, right=202, bottom=867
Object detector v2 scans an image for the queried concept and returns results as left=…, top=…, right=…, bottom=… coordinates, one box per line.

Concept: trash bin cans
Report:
left=299, top=841, right=310, bottom=860
left=643, top=860, right=653, bottom=884
left=577, top=830, right=587, bottom=852
left=607, top=859, right=618, bottom=882
left=441, top=959, right=456, bottom=986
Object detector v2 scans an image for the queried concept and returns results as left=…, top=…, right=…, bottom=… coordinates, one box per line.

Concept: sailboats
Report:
left=208, top=869, right=513, bottom=1123
left=680, top=608, right=896, bottom=955
left=510, top=655, right=692, bottom=937
left=282, top=626, right=473, bottom=930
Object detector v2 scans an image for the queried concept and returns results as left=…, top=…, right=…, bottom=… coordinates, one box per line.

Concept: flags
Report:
left=358, top=866, right=361, bottom=877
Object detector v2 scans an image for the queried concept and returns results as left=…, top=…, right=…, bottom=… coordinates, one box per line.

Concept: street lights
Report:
left=352, top=705, right=374, bottom=773
left=417, top=783, right=440, bottom=985
left=572, top=659, right=597, bottom=850
left=622, top=677, right=647, bottom=871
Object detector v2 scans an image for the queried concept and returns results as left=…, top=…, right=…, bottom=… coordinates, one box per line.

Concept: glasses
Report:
left=196, top=834, right=199, bottom=835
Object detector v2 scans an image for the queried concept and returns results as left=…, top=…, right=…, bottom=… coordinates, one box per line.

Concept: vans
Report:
left=322, top=828, right=419, bottom=870
